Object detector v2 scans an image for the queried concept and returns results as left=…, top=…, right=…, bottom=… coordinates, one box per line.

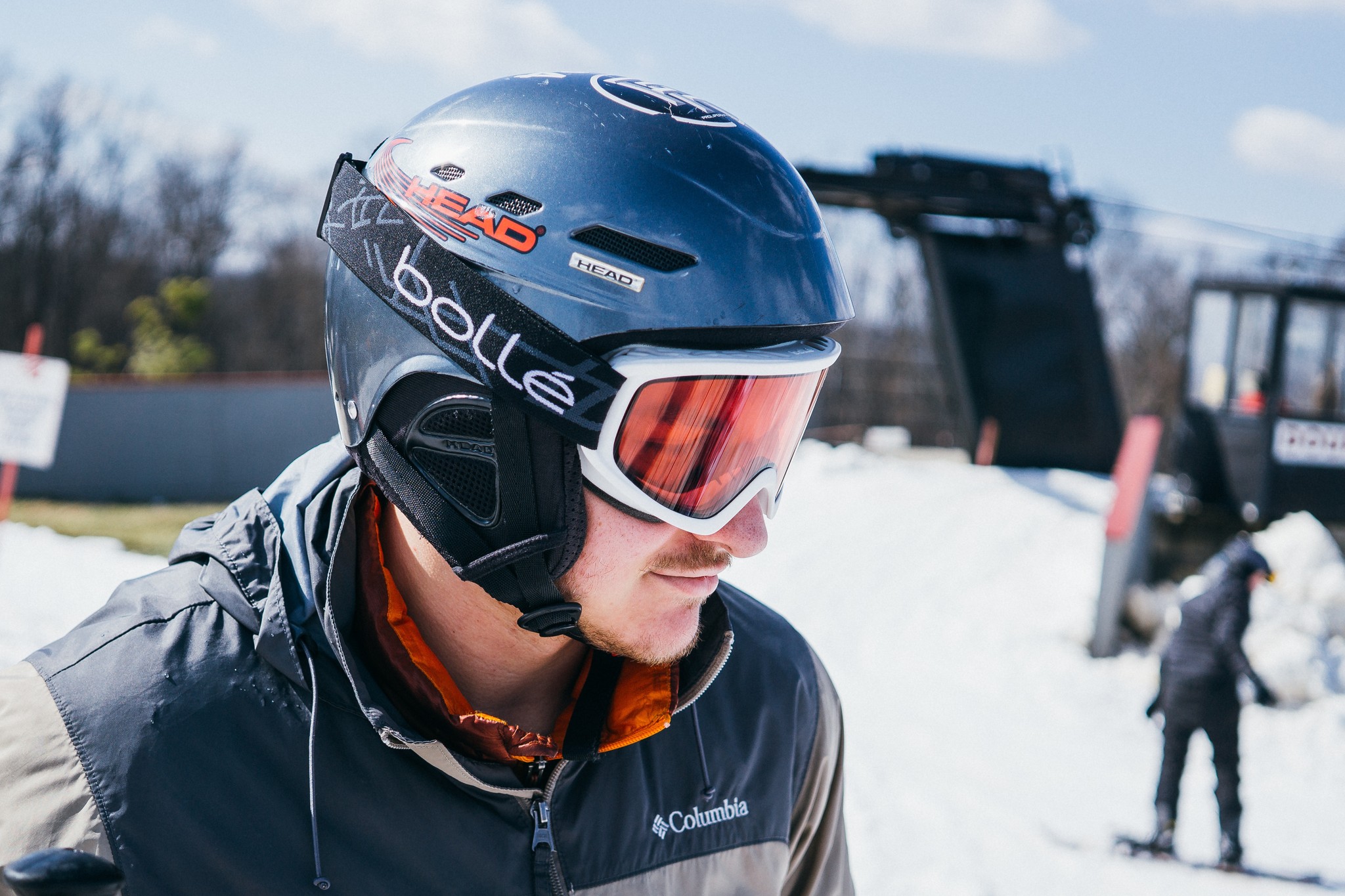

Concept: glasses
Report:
left=574, top=339, right=846, bottom=538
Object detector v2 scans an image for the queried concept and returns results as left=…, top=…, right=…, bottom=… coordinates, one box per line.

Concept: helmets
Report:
left=1229, top=551, right=1272, bottom=582
left=323, top=72, right=849, bottom=655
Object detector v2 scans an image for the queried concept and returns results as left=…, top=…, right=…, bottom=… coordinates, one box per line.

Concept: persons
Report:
left=1145, top=399, right=1279, bottom=718
left=4, top=74, right=856, bottom=894
left=1113, top=547, right=1280, bottom=874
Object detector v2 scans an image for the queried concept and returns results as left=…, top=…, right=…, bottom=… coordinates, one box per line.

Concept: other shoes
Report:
left=1151, top=817, right=1180, bottom=849
left=1218, top=834, right=1245, bottom=862
